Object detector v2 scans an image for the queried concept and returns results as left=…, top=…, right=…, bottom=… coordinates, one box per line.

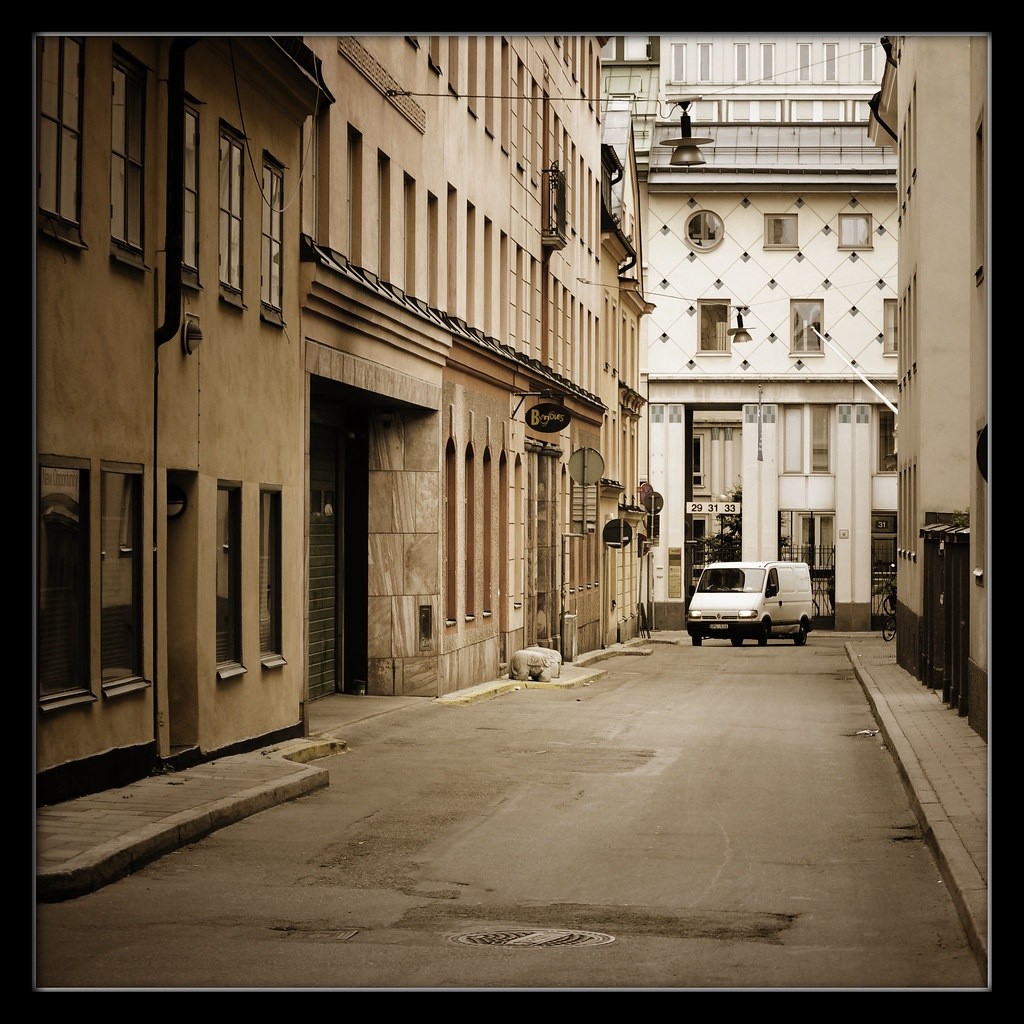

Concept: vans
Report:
left=686, top=560, right=815, bottom=646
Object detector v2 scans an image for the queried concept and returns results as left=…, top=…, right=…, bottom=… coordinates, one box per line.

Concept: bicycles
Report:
left=882, top=577, right=897, bottom=616
left=882, top=605, right=897, bottom=641
